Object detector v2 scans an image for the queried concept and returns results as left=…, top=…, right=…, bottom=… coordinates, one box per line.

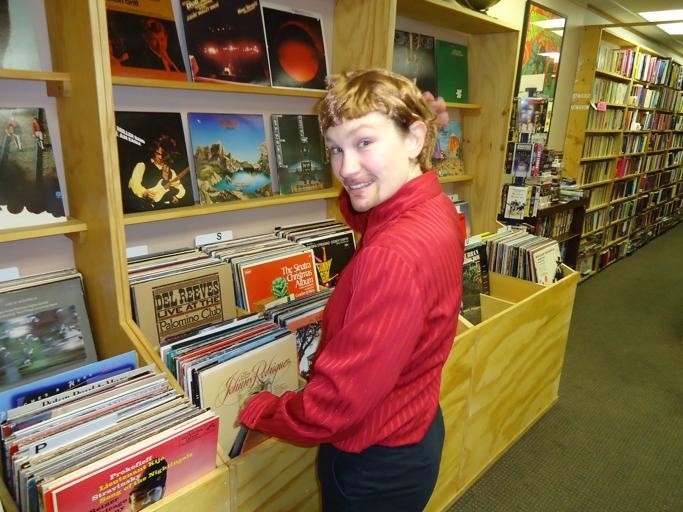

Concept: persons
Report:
left=232, top=66, right=469, bottom=511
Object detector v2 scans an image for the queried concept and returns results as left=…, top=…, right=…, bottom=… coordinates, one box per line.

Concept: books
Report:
left=482, top=48, right=682, bottom=286
left=0, top=0, right=363, bottom=511
left=394, top=28, right=436, bottom=105
left=430, top=118, right=464, bottom=179
left=434, top=38, right=469, bottom=106
left=459, top=237, right=490, bottom=311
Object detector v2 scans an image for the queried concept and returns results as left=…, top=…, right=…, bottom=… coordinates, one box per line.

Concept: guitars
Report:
left=148, top=167, right=190, bottom=202
